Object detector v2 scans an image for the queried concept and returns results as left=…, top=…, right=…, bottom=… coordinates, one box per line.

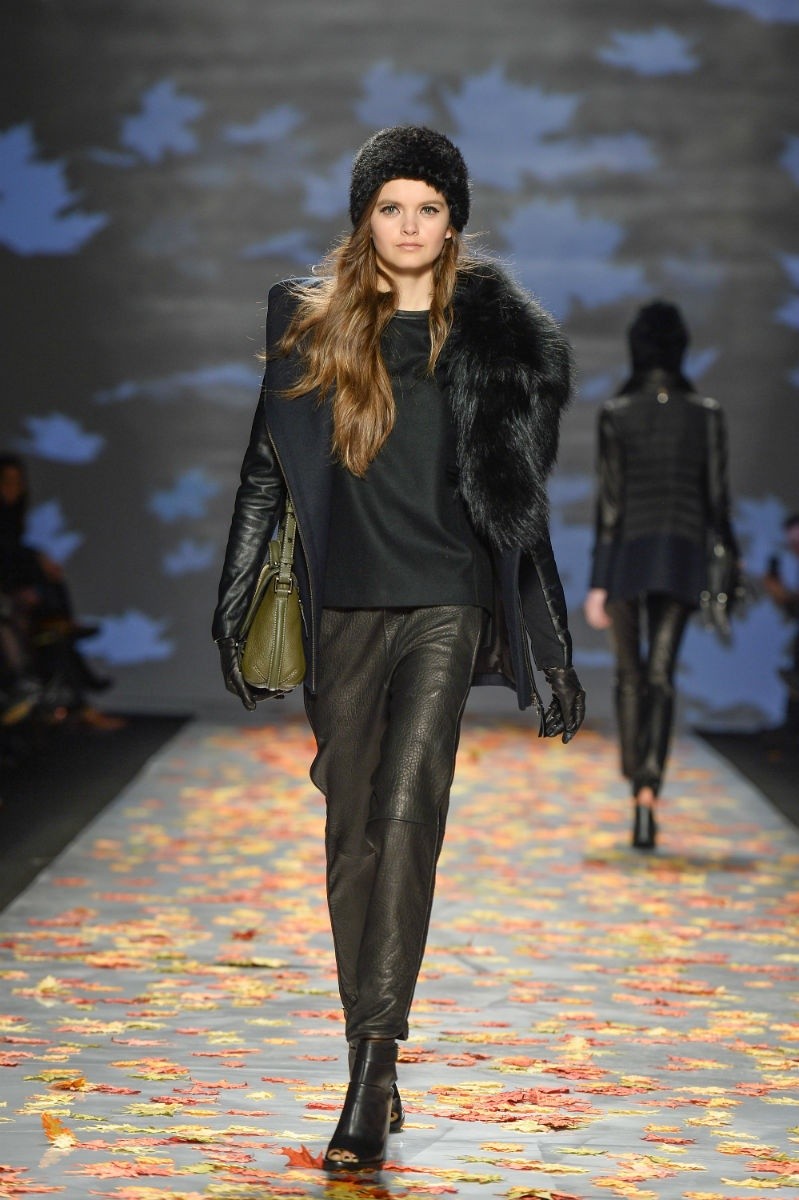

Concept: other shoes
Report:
left=633, top=804, right=655, bottom=850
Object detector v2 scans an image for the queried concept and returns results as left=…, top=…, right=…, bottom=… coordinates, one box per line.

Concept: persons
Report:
left=585, top=300, right=799, bottom=849
left=212, top=122, right=589, bottom=1172
left=1, top=462, right=128, bottom=740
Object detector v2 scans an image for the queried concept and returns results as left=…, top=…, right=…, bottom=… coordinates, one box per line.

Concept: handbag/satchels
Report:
left=705, top=529, right=758, bottom=643
left=241, top=490, right=306, bottom=693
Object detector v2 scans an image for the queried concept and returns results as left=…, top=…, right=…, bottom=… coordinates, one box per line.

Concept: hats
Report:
left=350, top=125, right=469, bottom=233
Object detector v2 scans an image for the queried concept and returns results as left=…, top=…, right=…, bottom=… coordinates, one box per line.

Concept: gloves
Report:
left=219, top=639, right=295, bottom=711
left=538, top=666, right=587, bottom=744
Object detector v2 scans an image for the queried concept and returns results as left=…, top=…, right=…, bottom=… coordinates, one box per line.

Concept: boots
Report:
left=322, top=1040, right=406, bottom=1172
